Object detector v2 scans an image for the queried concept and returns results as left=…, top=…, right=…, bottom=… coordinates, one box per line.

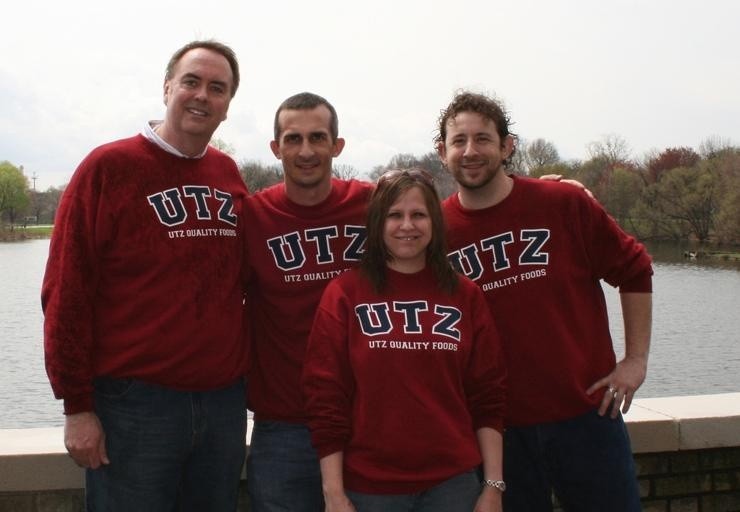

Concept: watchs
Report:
left=480, top=478, right=507, bottom=491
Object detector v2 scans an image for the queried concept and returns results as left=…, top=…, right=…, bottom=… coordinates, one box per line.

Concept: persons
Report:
left=38, top=38, right=252, bottom=512
left=434, top=90, right=655, bottom=510
left=297, top=165, right=513, bottom=511
left=240, top=90, right=596, bottom=511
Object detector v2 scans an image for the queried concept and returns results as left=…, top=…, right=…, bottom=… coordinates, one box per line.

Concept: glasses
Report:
left=370, top=169, right=434, bottom=200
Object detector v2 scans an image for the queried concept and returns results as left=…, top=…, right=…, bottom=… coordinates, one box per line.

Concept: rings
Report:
left=607, top=388, right=615, bottom=393
